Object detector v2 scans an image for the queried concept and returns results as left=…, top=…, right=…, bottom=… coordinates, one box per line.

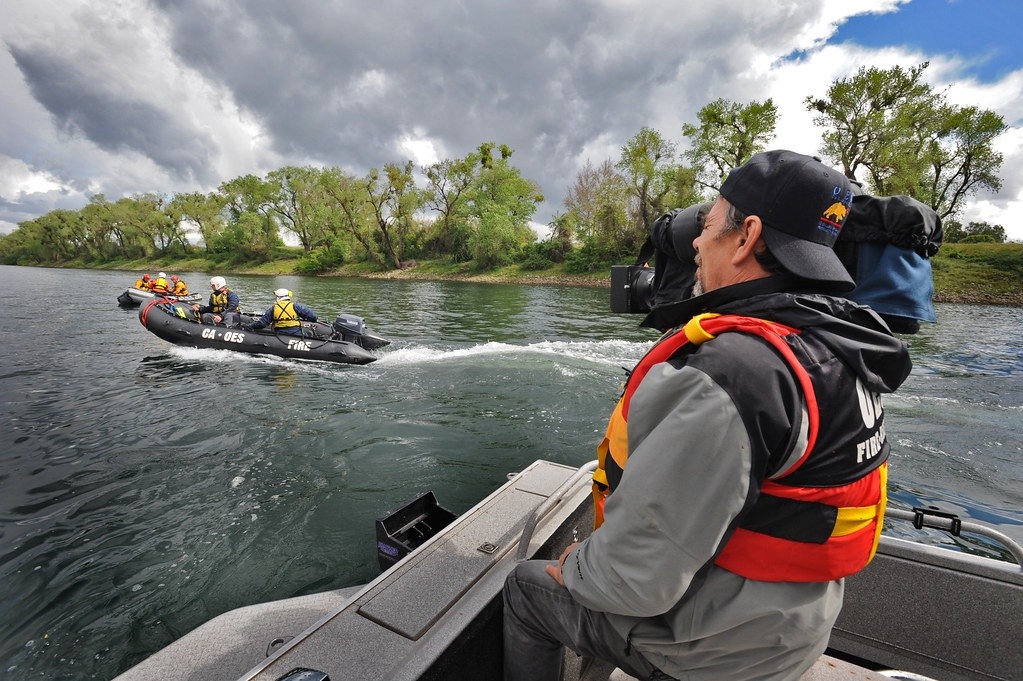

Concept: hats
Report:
left=719, top=150, right=856, bottom=292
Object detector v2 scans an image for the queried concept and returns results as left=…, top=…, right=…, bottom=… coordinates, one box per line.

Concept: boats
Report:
left=137, top=292, right=392, bottom=366
left=116, top=286, right=201, bottom=310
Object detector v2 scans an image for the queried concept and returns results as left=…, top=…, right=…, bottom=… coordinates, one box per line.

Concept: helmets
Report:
left=210, top=276, right=227, bottom=290
left=144, top=274, right=150, bottom=279
left=159, top=272, right=166, bottom=279
left=275, top=289, right=290, bottom=302
left=171, top=275, right=179, bottom=282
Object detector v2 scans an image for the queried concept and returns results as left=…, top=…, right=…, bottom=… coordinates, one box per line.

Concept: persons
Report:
left=135, top=274, right=152, bottom=292
left=150, top=272, right=170, bottom=295
left=171, top=275, right=188, bottom=296
left=247, top=288, right=319, bottom=338
left=503, top=150, right=912, bottom=681
left=194, top=277, right=240, bottom=329
